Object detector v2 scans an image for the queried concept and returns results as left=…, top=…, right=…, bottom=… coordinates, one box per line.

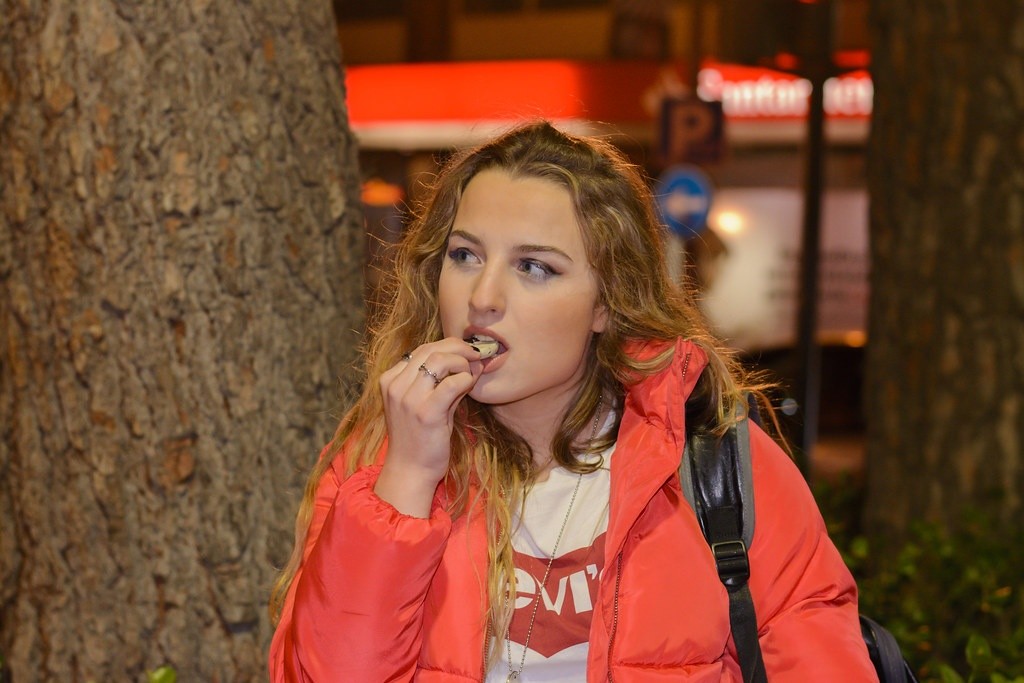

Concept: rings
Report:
left=418, top=362, right=442, bottom=387
left=403, top=352, right=413, bottom=363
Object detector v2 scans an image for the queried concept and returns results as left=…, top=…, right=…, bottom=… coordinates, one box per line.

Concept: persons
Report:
left=268, top=116, right=880, bottom=683
